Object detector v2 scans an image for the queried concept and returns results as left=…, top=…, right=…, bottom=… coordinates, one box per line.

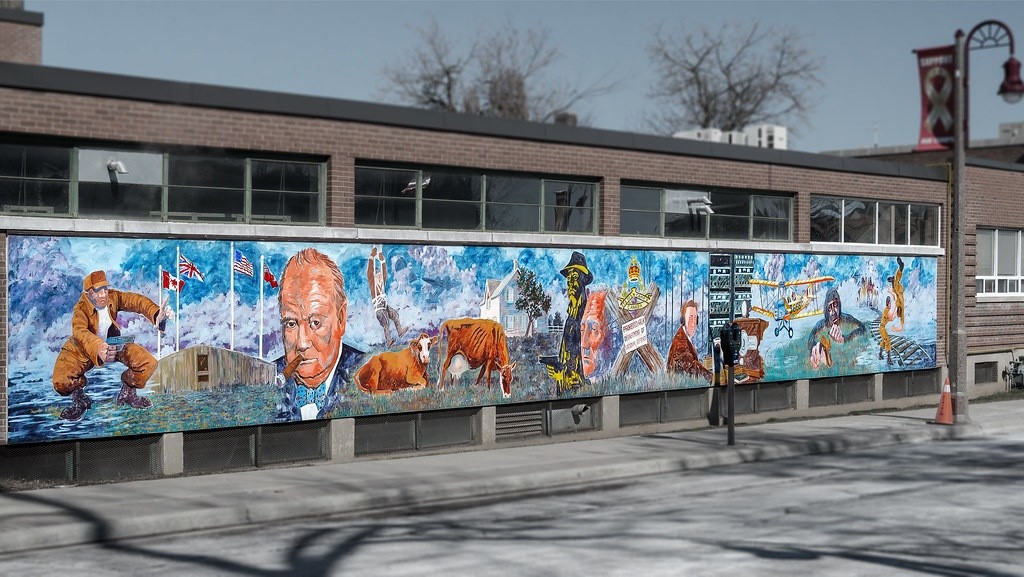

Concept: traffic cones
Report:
left=924, top=376, right=956, bottom=427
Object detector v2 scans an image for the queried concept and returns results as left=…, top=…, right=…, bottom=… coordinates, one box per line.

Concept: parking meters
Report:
left=718, top=322, right=743, bottom=447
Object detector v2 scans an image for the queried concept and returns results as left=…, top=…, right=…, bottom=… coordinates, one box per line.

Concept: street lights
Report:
left=949, top=20, right=1023, bottom=427
